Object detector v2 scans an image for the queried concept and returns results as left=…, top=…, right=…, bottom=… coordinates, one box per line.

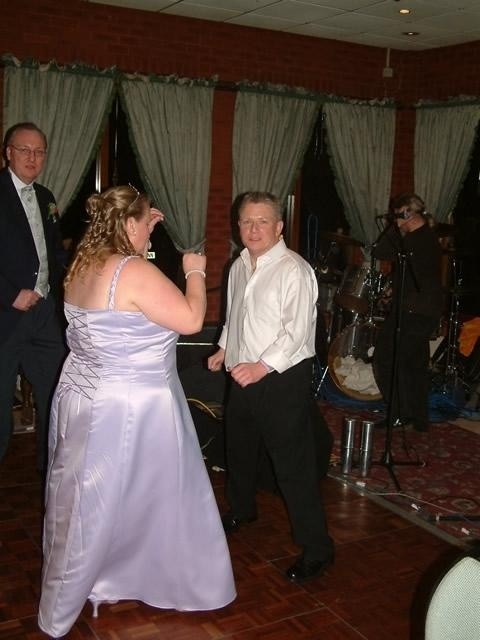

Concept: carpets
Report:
left=305, top=392, right=479, bottom=550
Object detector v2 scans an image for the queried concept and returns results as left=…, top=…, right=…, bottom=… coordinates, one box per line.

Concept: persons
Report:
left=0, top=122, right=71, bottom=482
left=35, top=184, right=239, bottom=640
left=374, top=192, right=445, bottom=435
left=207, top=188, right=337, bottom=583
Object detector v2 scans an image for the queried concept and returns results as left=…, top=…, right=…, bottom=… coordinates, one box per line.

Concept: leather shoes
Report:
left=287, top=538, right=333, bottom=583
left=221, top=502, right=259, bottom=532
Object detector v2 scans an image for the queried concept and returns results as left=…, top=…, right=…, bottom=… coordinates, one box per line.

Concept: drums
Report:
left=327, top=316, right=385, bottom=400
left=336, top=265, right=392, bottom=314
left=316, top=268, right=342, bottom=312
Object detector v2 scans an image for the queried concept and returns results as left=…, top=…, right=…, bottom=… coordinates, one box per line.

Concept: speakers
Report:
left=176, top=324, right=232, bottom=473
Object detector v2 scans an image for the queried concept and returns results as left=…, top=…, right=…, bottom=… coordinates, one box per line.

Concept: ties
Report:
left=21, top=185, right=50, bottom=301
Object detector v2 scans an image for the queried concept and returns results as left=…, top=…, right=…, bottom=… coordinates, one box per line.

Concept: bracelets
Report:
left=183, top=267, right=206, bottom=279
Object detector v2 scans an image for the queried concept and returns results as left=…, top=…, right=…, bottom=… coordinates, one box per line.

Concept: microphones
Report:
left=384, top=211, right=410, bottom=220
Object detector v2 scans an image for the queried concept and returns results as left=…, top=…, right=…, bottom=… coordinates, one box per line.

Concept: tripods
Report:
left=432, top=255, right=480, bottom=396
left=328, top=220, right=428, bottom=498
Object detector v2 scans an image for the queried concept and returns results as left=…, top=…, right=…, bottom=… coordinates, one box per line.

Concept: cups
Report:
left=338, top=479, right=369, bottom=527
left=358, top=448, right=372, bottom=479
left=340, top=414, right=357, bottom=449
left=359, top=419, right=375, bottom=454
left=339, top=445, right=353, bottom=476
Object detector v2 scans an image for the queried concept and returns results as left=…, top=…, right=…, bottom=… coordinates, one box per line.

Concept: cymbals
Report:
left=320, top=231, right=366, bottom=247
left=434, top=223, right=461, bottom=237
